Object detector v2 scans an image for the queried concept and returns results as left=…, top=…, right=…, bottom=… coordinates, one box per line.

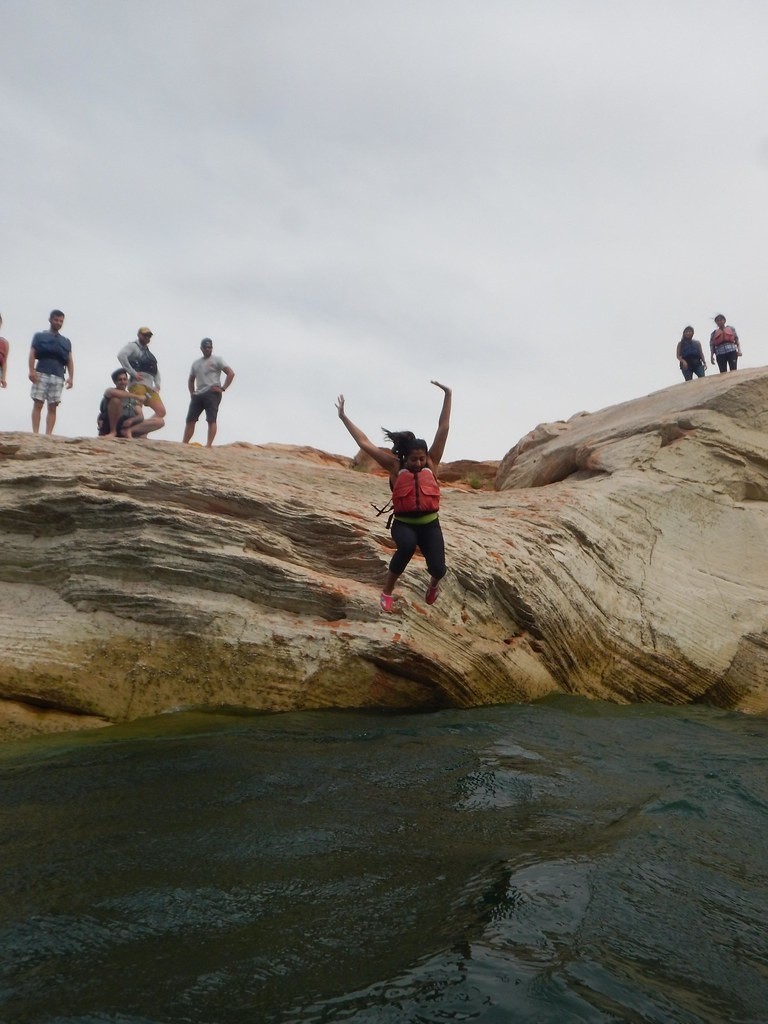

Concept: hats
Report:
left=138, top=326, right=153, bottom=335
left=202, top=338, right=213, bottom=348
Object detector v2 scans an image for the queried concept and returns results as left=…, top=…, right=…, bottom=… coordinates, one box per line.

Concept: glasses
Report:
left=142, top=333, right=151, bottom=338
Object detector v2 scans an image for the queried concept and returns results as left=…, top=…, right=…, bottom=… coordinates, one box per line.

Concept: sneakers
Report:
left=424, top=576, right=441, bottom=606
left=378, top=589, right=393, bottom=612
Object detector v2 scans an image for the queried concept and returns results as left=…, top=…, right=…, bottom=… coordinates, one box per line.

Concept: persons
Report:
left=182, top=338, right=235, bottom=447
left=97, top=369, right=165, bottom=439
left=29, top=310, right=74, bottom=435
left=335, top=380, right=452, bottom=611
left=0, top=313, right=9, bottom=388
left=676, top=327, right=708, bottom=381
left=710, top=315, right=743, bottom=375
left=117, top=327, right=167, bottom=437
